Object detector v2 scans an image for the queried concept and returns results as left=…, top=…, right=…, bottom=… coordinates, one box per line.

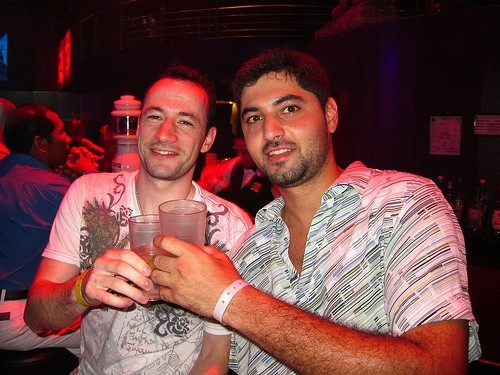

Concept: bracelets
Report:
left=213, top=279, right=249, bottom=325
left=75, top=268, right=93, bottom=306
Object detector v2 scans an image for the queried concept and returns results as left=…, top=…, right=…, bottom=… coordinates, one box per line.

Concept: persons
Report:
left=211, top=134, right=281, bottom=218
left=151, top=50, right=481, bottom=375
left=0, top=99, right=115, bottom=358
left=25, top=68, right=252, bottom=375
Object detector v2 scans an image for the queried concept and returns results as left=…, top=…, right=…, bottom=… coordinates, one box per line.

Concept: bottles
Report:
left=111, top=96, right=142, bottom=136
left=112, top=133, right=141, bottom=173
left=437, top=176, right=500, bottom=239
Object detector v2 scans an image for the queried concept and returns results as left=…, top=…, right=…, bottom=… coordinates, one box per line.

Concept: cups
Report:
left=128, top=214, right=163, bottom=302
left=159, top=199, right=207, bottom=247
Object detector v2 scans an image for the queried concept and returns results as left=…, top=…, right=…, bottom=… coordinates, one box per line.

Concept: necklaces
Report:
left=136, top=176, right=191, bottom=214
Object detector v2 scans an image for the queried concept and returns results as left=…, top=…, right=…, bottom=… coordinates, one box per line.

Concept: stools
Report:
left=0, top=347, right=79, bottom=375
